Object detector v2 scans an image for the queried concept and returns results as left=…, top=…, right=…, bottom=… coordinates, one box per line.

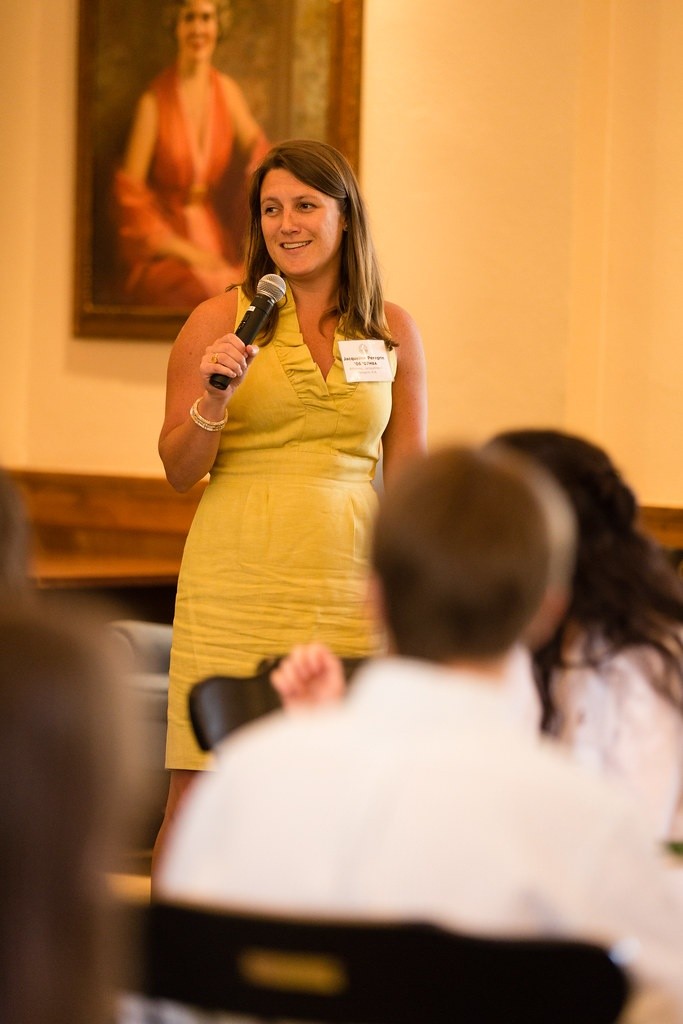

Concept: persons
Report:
left=478, top=428, right=683, bottom=716
left=149, top=443, right=683, bottom=1024
left=0, top=606, right=213, bottom=1024
left=149, top=138, right=427, bottom=881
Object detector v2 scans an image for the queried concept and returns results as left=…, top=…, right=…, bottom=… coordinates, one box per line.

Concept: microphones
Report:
left=207, top=274, right=287, bottom=390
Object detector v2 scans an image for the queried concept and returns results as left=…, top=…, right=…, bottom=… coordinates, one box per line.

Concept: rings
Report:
left=211, top=353, right=219, bottom=363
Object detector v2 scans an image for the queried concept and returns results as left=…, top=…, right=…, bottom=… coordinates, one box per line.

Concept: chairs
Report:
left=104, top=620, right=635, bottom=1024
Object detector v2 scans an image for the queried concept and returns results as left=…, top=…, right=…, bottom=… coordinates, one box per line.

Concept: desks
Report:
left=31, top=556, right=182, bottom=587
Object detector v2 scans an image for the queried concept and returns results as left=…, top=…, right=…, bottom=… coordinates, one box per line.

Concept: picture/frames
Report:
left=71, top=0, right=366, bottom=341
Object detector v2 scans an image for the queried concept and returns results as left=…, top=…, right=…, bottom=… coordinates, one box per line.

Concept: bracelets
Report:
left=190, top=397, right=228, bottom=432
